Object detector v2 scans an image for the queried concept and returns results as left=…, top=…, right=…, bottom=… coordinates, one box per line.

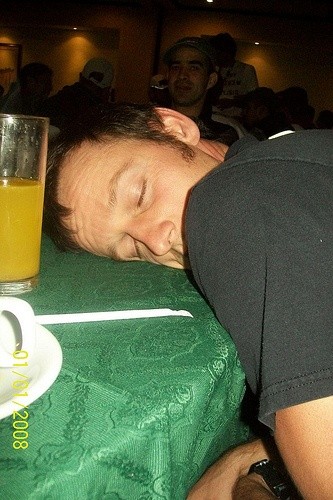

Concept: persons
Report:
left=164, top=36, right=254, bottom=151
left=234, top=86, right=333, bottom=141
left=4, top=62, right=62, bottom=125
left=36, top=94, right=333, bottom=500
left=60, top=55, right=118, bottom=116
left=211, top=31, right=262, bottom=117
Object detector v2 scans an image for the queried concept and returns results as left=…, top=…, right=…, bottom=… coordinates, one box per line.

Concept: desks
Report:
left=0, top=225, right=244, bottom=500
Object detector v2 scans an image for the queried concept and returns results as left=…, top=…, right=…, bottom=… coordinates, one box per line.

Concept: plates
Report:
left=0, top=322, right=63, bottom=420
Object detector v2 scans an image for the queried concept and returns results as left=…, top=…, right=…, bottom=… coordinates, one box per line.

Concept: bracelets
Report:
left=246, top=455, right=301, bottom=500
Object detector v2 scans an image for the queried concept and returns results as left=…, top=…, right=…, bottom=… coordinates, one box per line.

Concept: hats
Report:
left=81, top=57, right=113, bottom=91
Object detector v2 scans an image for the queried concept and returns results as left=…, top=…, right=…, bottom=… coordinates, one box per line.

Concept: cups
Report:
left=0, top=113, right=49, bottom=295
left=0, top=297, right=35, bottom=367
